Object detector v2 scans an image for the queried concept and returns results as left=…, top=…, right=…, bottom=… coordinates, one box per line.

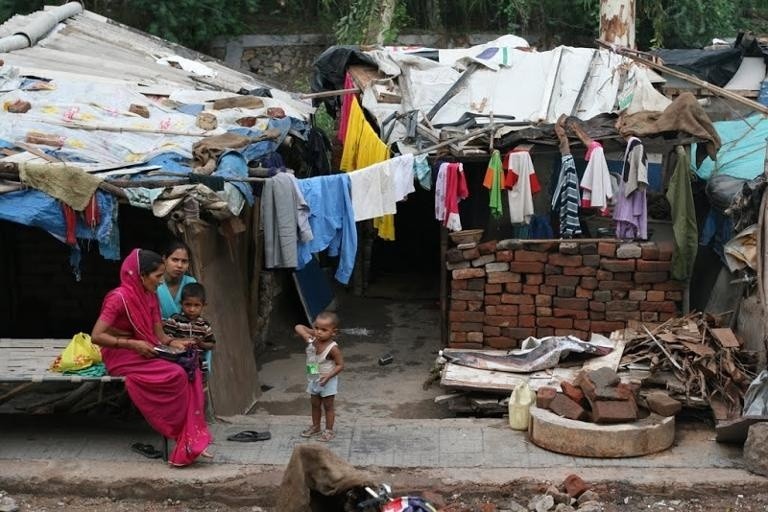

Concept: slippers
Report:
left=227, top=430, right=271, bottom=442
left=131, top=443, right=163, bottom=458
left=301, top=426, right=335, bottom=442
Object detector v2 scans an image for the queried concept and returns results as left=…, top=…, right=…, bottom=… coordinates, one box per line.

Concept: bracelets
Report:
left=165, top=338, right=176, bottom=346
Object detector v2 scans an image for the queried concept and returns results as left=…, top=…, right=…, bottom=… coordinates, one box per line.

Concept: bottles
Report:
left=305, top=338, right=321, bottom=384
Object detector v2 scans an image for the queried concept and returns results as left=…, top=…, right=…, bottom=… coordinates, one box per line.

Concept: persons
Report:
left=89, top=247, right=215, bottom=468
left=162, top=282, right=220, bottom=416
left=156, top=242, right=212, bottom=375
left=294, top=310, right=346, bottom=441
